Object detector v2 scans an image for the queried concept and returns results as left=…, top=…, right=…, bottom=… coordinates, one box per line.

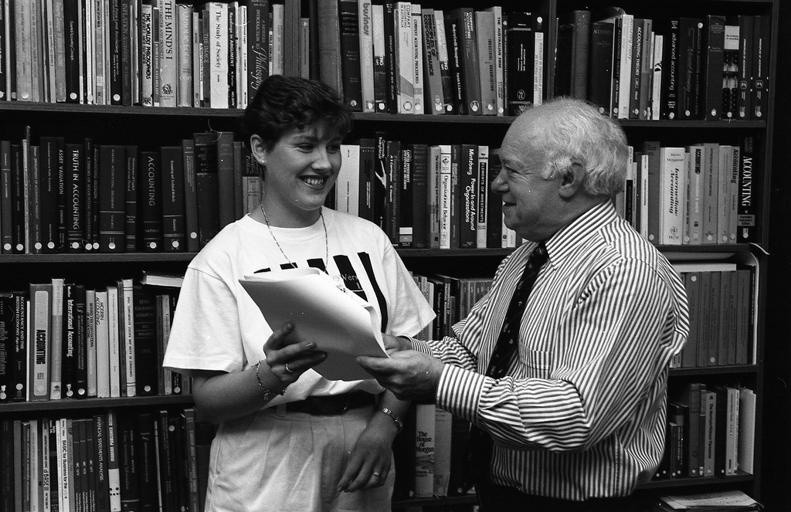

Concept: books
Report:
left=403, top=404, right=475, bottom=498
left=235, top=264, right=392, bottom=382
left=550, top=1, right=631, bottom=123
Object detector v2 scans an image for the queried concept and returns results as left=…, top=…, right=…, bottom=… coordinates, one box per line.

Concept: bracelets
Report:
left=381, top=406, right=404, bottom=429
left=255, top=361, right=288, bottom=400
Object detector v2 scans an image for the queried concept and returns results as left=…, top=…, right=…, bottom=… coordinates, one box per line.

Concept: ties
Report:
left=484, top=241, right=551, bottom=378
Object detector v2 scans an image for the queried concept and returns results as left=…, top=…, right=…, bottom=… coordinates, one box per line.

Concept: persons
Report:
left=354, top=93, right=693, bottom=511
left=159, top=73, right=443, bottom=512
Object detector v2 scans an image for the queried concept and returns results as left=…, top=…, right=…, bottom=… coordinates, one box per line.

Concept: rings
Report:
left=285, top=363, right=295, bottom=374
left=371, top=470, right=382, bottom=479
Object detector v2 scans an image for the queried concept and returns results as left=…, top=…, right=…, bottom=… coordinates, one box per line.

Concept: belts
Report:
left=287, top=389, right=375, bottom=416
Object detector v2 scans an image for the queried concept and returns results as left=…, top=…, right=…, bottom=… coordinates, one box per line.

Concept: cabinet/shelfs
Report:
left=1, top=1, right=782, bottom=511
